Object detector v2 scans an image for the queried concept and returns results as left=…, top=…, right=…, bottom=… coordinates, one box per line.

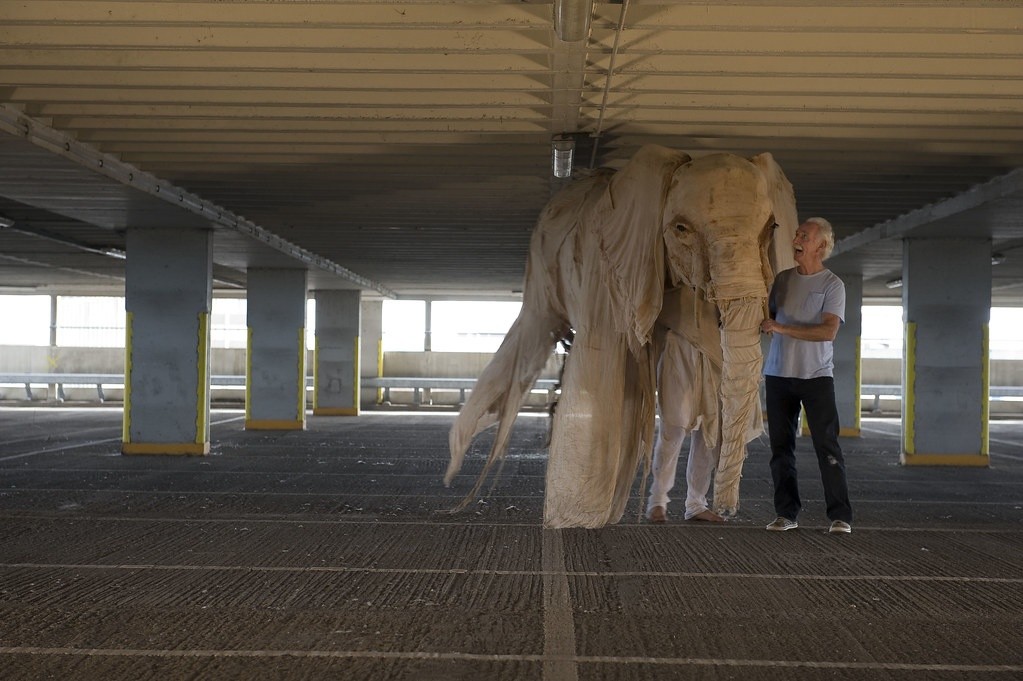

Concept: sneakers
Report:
left=829, top=520, right=851, bottom=534
left=766, top=517, right=797, bottom=531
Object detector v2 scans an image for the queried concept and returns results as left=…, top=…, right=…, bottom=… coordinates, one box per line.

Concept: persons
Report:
left=647, top=332, right=721, bottom=523
left=757, top=218, right=855, bottom=535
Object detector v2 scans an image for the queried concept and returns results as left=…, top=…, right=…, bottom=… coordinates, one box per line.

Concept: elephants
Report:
left=441, top=145, right=800, bottom=530
